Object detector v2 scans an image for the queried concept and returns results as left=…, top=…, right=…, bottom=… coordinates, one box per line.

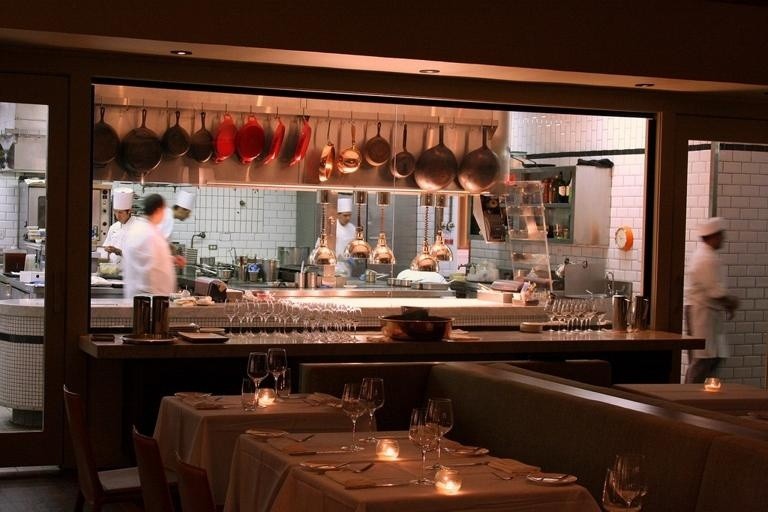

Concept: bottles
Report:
left=132, top=296, right=150, bottom=336
left=524, top=171, right=572, bottom=203
left=152, top=295, right=171, bottom=339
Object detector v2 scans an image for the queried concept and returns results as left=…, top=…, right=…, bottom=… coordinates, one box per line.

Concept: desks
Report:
left=613, top=380, right=767, bottom=411
left=79, top=325, right=706, bottom=402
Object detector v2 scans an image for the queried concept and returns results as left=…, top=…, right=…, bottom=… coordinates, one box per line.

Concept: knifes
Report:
left=447, top=462, right=492, bottom=468
left=288, top=449, right=356, bottom=457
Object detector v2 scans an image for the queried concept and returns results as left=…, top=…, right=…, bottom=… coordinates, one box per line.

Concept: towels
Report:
left=486, top=456, right=540, bottom=475
left=322, top=467, right=374, bottom=489
left=183, top=394, right=221, bottom=408
left=266, top=434, right=306, bottom=454
left=308, top=390, right=336, bottom=403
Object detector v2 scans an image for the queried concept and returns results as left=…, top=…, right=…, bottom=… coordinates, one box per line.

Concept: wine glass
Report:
left=225, top=296, right=362, bottom=343
left=544, top=294, right=608, bottom=334
left=341, top=377, right=454, bottom=488
left=597, top=448, right=655, bottom=512
left=246, top=348, right=286, bottom=408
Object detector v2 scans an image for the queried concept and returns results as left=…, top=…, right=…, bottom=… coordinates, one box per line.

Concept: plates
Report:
left=527, top=470, right=579, bottom=485
left=122, top=332, right=178, bottom=347
left=173, top=322, right=227, bottom=343
left=174, top=391, right=212, bottom=399
left=301, top=462, right=336, bottom=473
left=447, top=445, right=492, bottom=458
left=246, top=426, right=286, bottom=438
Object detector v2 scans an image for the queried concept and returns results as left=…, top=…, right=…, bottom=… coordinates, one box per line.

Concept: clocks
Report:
left=615, top=225, right=634, bottom=252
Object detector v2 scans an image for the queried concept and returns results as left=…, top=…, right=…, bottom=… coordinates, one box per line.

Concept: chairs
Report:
left=173, top=450, right=217, bottom=509
left=129, top=425, right=174, bottom=510
left=62, top=381, right=180, bottom=509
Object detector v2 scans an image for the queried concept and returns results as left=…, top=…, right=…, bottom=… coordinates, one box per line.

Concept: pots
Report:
left=92, top=105, right=500, bottom=192
left=277, top=246, right=311, bottom=268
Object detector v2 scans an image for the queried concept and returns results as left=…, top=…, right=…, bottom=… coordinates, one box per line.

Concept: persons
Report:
left=96, top=208, right=137, bottom=272
left=682, top=227, right=739, bottom=385
left=315, top=212, right=357, bottom=260
left=121, top=194, right=188, bottom=299
left=153, top=203, right=190, bottom=251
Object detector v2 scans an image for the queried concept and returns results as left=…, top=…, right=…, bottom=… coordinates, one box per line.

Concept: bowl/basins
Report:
left=26, top=225, right=48, bottom=244
left=198, top=255, right=261, bottom=282
left=98, top=263, right=120, bottom=279
left=377, top=316, right=456, bottom=339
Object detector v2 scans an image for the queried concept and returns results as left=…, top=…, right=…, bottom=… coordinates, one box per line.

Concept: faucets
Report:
left=457, top=263, right=473, bottom=277
left=604, top=271, right=616, bottom=295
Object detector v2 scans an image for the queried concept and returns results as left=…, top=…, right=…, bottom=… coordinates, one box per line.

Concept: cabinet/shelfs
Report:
left=467, top=164, right=613, bottom=249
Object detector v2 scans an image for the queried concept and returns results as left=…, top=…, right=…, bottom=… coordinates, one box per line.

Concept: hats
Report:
left=698, top=216, right=723, bottom=235
left=337, top=198, right=352, bottom=212
left=175, top=190, right=196, bottom=212
left=113, top=187, right=134, bottom=210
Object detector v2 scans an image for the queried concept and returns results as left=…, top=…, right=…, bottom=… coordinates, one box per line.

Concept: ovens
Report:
left=17, top=187, right=105, bottom=242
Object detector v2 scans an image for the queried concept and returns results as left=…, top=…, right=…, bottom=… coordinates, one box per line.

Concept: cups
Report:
left=295, top=272, right=318, bottom=286
left=242, top=375, right=261, bottom=412
left=554, top=222, right=570, bottom=240
left=704, top=377, right=721, bottom=392
left=276, top=368, right=293, bottom=397
left=1, top=249, right=45, bottom=275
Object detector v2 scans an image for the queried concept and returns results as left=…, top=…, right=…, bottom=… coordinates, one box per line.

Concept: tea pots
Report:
left=610, top=293, right=650, bottom=331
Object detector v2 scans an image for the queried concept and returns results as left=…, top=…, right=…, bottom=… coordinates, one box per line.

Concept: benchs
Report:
left=301, top=361, right=766, bottom=509
left=476, top=356, right=767, bottom=432
